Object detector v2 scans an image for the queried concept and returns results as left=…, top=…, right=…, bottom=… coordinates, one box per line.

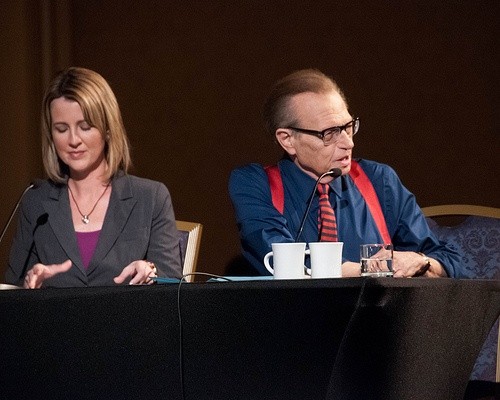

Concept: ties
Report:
left=317, top=183, right=338, bottom=242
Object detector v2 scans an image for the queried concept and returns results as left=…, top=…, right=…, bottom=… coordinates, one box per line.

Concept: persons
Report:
left=227, top=69, right=474, bottom=278
left=0, top=67, right=185, bottom=290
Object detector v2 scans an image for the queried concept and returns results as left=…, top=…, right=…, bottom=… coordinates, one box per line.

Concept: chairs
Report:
left=420, top=204, right=500, bottom=384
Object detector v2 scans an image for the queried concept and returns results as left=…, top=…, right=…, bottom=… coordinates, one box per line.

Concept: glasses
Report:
left=287, top=117, right=360, bottom=146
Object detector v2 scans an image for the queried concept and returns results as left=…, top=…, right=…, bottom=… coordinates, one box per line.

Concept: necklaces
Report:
left=69, top=181, right=111, bottom=224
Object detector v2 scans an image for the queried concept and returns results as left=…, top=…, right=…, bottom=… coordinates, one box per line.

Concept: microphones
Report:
left=0, top=178, right=42, bottom=245
left=296, top=168, right=342, bottom=242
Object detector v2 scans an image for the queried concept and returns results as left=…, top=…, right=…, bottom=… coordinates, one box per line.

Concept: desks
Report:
left=0, top=277, right=500, bottom=400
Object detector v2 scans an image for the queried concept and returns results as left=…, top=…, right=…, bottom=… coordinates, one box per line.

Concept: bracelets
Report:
left=150, top=263, right=157, bottom=274
left=419, top=253, right=429, bottom=264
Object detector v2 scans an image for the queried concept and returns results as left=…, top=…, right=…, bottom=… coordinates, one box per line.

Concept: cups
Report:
left=264, top=243, right=306, bottom=279
left=360, top=243, right=394, bottom=277
left=304, top=242, right=343, bottom=278
